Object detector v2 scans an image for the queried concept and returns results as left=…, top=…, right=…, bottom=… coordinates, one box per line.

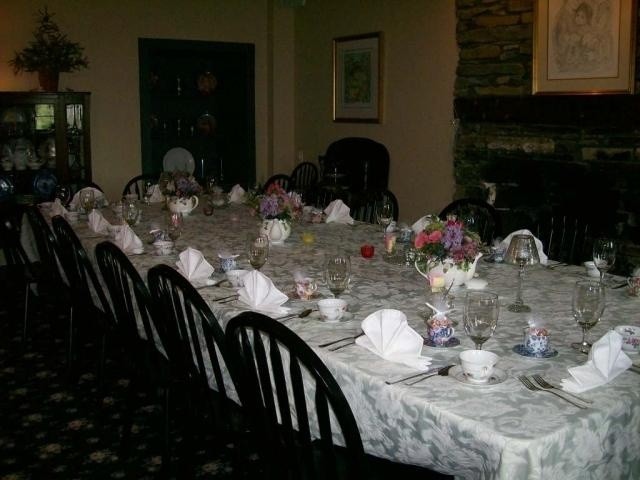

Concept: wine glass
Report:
left=570, top=278, right=606, bottom=352
left=592, top=238, right=616, bottom=284
left=56, top=185, right=70, bottom=207
left=167, top=213, right=184, bottom=240
left=246, top=235, right=269, bottom=269
left=79, top=189, right=94, bottom=215
left=504, top=234, right=541, bottom=313
left=327, top=254, right=351, bottom=298
left=158, top=173, right=176, bottom=210
left=205, top=176, right=218, bottom=198
left=462, top=291, right=500, bottom=350
left=142, top=180, right=155, bottom=207
left=376, top=196, right=394, bottom=239
left=121, top=199, right=140, bottom=226
left=247, top=181, right=261, bottom=203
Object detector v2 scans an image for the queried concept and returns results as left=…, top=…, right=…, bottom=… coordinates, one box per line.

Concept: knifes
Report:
left=274, top=309, right=318, bottom=320
left=387, top=366, right=443, bottom=385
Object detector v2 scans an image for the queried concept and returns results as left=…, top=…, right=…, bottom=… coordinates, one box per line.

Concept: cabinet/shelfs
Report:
left=0, top=91, right=92, bottom=272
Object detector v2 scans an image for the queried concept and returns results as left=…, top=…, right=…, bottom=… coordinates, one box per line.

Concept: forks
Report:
left=319, top=333, right=364, bottom=351
left=517, top=373, right=594, bottom=411
left=213, top=294, right=240, bottom=304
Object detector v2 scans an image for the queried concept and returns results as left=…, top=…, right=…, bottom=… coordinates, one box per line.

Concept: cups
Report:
left=317, top=298, right=347, bottom=324
left=458, top=350, right=501, bottom=381
left=426, top=318, right=455, bottom=347
left=218, top=253, right=241, bottom=274
left=523, top=327, right=550, bottom=354
left=294, top=277, right=318, bottom=301
left=584, top=261, right=605, bottom=279
left=154, top=242, right=174, bottom=256
left=627, top=276, right=640, bottom=296
left=614, top=325, right=640, bottom=354
left=490, top=246, right=506, bottom=263
left=400, top=229, right=412, bottom=243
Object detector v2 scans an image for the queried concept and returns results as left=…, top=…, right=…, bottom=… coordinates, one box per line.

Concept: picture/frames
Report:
left=530, top=0, right=638, bottom=97
left=332, top=31, right=386, bottom=124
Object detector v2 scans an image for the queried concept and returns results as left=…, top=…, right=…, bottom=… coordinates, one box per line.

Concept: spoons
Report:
left=404, top=365, right=456, bottom=387
left=280, top=308, right=313, bottom=324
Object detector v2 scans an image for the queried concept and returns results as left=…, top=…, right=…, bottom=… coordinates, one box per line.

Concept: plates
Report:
left=448, top=363, right=509, bottom=388
left=5, top=139, right=37, bottom=159
left=514, top=344, right=558, bottom=357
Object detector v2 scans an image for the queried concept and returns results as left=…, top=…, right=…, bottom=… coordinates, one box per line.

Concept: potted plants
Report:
left=6, top=4, right=92, bottom=92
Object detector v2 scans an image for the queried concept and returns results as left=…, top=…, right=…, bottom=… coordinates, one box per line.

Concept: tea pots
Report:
left=3, top=143, right=27, bottom=170
